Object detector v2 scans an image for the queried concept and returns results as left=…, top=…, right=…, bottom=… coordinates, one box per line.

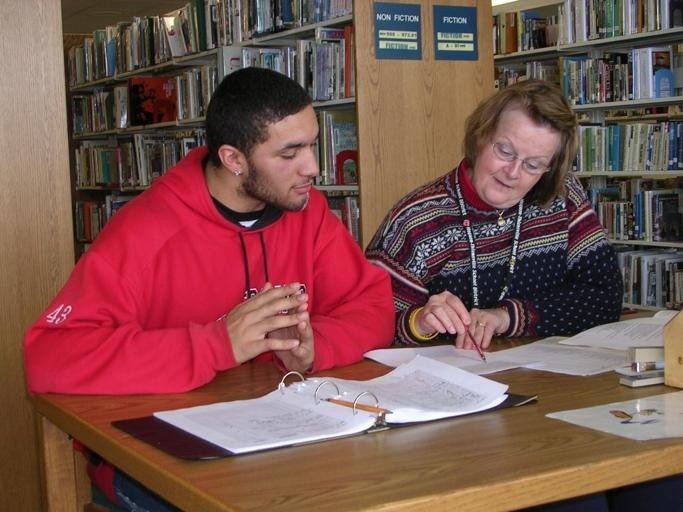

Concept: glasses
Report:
left=486, top=140, right=553, bottom=177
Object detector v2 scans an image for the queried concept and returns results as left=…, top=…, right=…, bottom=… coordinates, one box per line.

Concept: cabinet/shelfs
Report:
left=61, top=0, right=495, bottom=315
left=492, top=0, right=683, bottom=310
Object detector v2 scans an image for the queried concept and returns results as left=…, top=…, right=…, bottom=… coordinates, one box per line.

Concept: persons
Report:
left=359, top=76, right=624, bottom=349
left=19, top=69, right=395, bottom=510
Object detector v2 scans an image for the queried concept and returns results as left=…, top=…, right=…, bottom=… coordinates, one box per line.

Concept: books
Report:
left=108, top=356, right=539, bottom=462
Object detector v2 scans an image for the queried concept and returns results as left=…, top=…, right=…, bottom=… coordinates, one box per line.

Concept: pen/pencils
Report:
left=327, top=398, right=393, bottom=414
left=466, top=327, right=485, bottom=360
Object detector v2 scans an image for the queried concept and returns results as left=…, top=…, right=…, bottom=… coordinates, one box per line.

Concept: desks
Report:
left=31, top=311, right=682, bottom=512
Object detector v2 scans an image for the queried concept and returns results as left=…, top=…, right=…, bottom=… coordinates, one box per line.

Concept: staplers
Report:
left=619, top=346, right=665, bottom=388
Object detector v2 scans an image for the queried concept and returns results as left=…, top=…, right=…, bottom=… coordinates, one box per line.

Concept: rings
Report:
left=476, top=321, right=485, bottom=327
